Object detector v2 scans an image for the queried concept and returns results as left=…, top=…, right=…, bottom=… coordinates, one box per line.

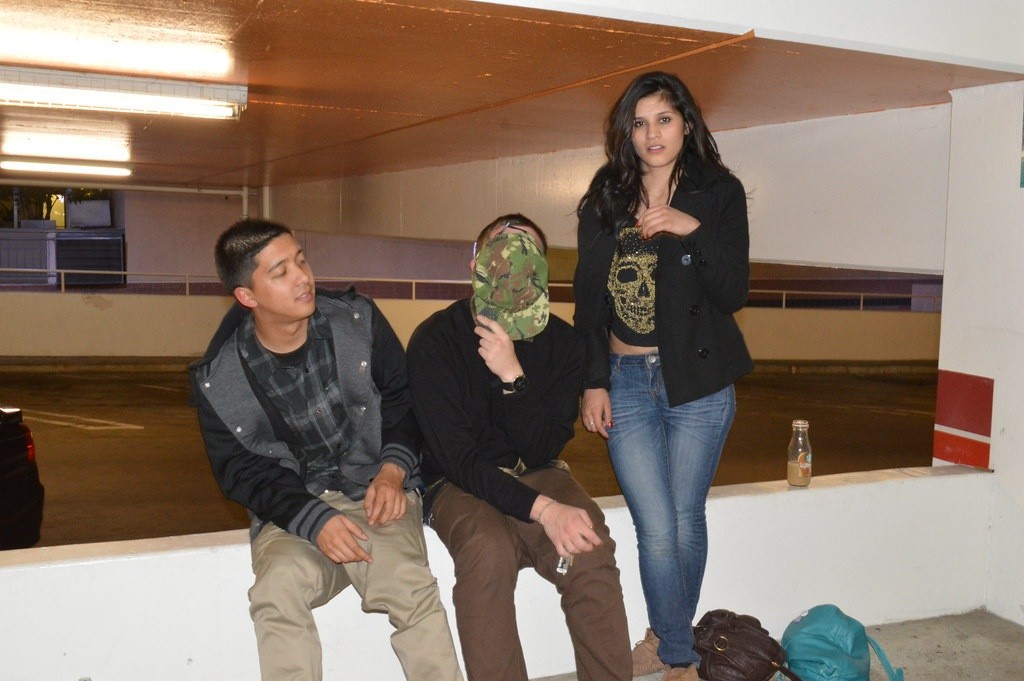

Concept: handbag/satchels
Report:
left=692, top=609, right=801, bottom=681
left=782, top=603, right=903, bottom=681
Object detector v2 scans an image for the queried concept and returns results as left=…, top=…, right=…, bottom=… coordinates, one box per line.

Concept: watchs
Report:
left=502, top=374, right=528, bottom=393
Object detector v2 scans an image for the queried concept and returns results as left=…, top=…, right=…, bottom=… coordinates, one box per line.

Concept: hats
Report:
left=472, top=226, right=550, bottom=342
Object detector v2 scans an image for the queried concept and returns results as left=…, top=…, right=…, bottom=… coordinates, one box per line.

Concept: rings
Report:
left=589, top=421, right=595, bottom=426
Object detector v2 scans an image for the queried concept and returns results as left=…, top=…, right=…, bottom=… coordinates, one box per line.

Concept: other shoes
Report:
left=630, top=628, right=668, bottom=676
left=661, top=661, right=699, bottom=680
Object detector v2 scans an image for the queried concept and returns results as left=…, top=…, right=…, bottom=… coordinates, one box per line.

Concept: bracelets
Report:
left=537, top=500, right=557, bottom=524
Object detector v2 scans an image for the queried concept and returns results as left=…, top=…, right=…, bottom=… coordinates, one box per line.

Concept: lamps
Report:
left=0, top=65, right=248, bottom=121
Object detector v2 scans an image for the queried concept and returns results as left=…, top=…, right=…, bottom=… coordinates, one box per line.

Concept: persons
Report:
left=573, top=71, right=754, bottom=681
left=193, top=219, right=465, bottom=681
left=407, top=213, right=634, bottom=681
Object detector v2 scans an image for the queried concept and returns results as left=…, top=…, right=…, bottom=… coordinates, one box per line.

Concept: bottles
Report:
left=787, top=420, right=812, bottom=486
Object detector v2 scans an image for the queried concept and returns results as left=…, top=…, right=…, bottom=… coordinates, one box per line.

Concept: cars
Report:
left=0, top=408, right=44, bottom=551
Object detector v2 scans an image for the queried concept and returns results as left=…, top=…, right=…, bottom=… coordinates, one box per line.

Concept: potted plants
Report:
left=0, top=184, right=58, bottom=229
left=60, top=187, right=112, bottom=228
